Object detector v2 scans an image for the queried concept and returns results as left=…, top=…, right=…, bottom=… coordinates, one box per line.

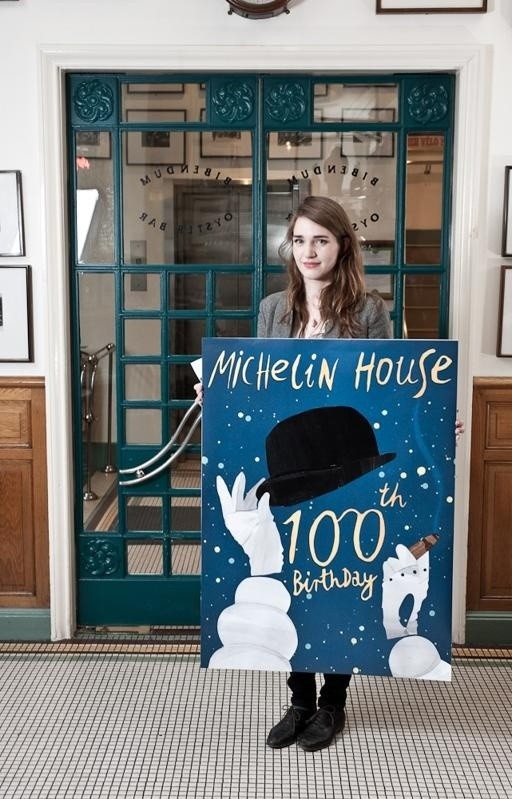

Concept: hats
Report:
left=256, top=406, right=396, bottom=507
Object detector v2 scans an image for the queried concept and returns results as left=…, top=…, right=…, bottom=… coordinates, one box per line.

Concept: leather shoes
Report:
left=267, top=705, right=346, bottom=752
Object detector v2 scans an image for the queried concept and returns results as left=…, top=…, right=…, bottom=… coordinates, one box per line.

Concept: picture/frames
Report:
left=0, top=167, right=38, bottom=365
left=124, top=81, right=400, bottom=167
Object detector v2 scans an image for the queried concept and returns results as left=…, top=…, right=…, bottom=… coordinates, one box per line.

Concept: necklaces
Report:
left=306, top=304, right=322, bottom=328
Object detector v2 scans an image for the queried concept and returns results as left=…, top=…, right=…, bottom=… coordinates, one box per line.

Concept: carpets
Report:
left=107, top=504, right=202, bottom=546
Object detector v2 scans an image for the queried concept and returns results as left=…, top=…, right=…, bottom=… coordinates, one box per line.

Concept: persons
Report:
left=196, top=197, right=465, bottom=752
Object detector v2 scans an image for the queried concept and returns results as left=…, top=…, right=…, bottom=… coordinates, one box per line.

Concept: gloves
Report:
left=381, top=544, right=429, bottom=640
left=215, top=472, right=284, bottom=576
